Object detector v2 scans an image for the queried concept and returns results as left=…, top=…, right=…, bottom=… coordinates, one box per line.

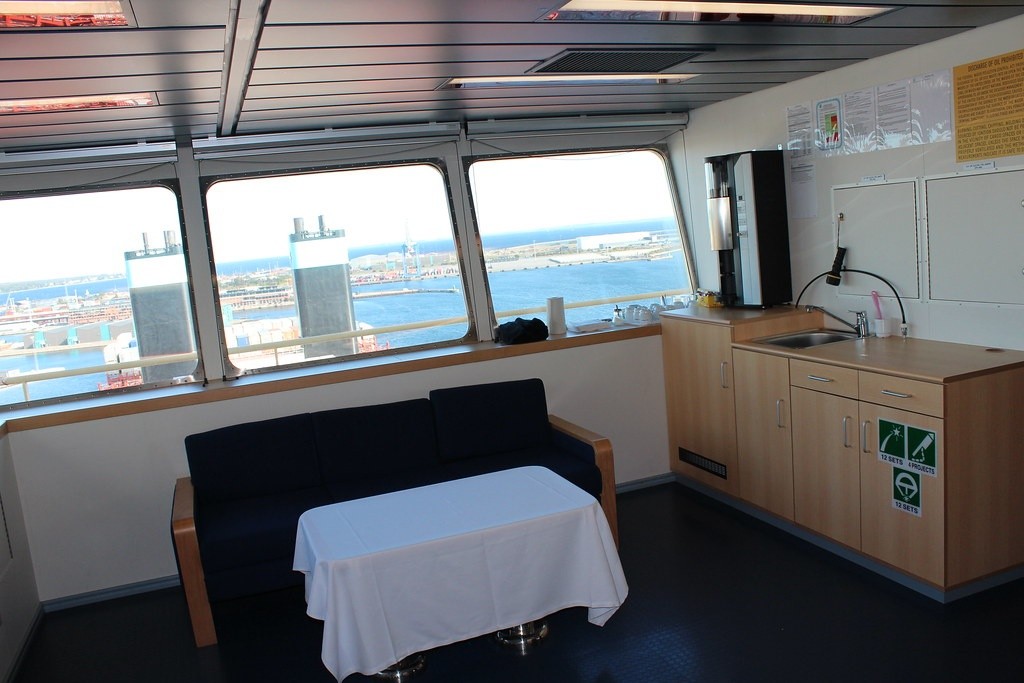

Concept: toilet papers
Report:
left=546, top=296, right=566, bottom=334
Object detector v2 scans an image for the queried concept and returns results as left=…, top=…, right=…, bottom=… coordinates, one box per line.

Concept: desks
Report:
left=293, top=466, right=628, bottom=683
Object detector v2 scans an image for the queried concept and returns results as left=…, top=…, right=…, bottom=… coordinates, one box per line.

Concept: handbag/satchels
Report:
left=494, top=318, right=549, bottom=345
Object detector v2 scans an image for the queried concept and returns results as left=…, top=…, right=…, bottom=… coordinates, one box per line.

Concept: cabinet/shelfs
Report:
left=659, top=303, right=1023, bottom=603
left=705, top=150, right=793, bottom=310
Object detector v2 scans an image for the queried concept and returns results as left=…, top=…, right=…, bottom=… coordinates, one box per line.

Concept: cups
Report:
left=639, top=308, right=652, bottom=323
left=651, top=306, right=663, bottom=321
left=875, top=319, right=892, bottom=338
left=633, top=308, right=641, bottom=321
left=666, top=305, right=675, bottom=311
left=674, top=302, right=684, bottom=309
left=625, top=308, right=633, bottom=320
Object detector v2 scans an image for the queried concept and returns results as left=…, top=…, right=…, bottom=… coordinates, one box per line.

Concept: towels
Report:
left=567, top=319, right=611, bottom=333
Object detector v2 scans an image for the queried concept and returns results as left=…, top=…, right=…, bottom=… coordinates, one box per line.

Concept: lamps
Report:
left=708, top=197, right=734, bottom=250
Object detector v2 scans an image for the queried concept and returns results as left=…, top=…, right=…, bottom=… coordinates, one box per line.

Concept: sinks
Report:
left=753, top=327, right=858, bottom=350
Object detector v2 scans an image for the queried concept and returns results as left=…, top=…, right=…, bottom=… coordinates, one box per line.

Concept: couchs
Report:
left=170, top=378, right=618, bottom=649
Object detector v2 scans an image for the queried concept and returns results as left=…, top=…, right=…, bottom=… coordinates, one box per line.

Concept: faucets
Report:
left=804, top=304, right=869, bottom=338
